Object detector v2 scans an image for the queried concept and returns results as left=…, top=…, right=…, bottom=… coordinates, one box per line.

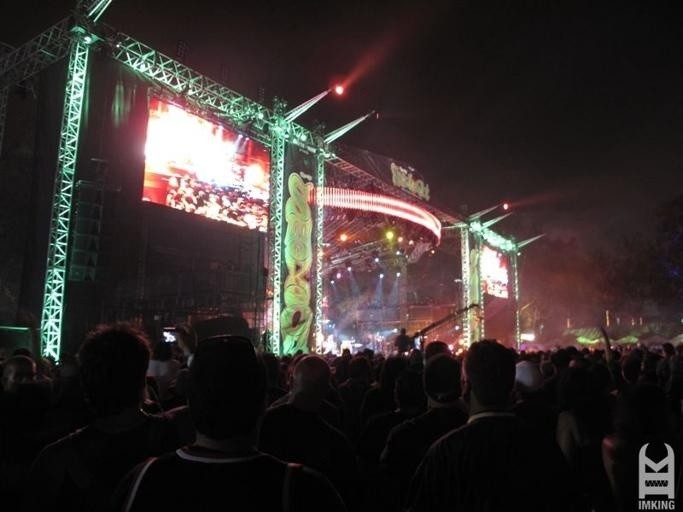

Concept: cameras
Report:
left=161, top=327, right=177, bottom=344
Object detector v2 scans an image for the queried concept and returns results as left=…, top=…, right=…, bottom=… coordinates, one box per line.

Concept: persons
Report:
left=163, top=171, right=269, bottom=234
left=1, top=326, right=683, bottom=510
